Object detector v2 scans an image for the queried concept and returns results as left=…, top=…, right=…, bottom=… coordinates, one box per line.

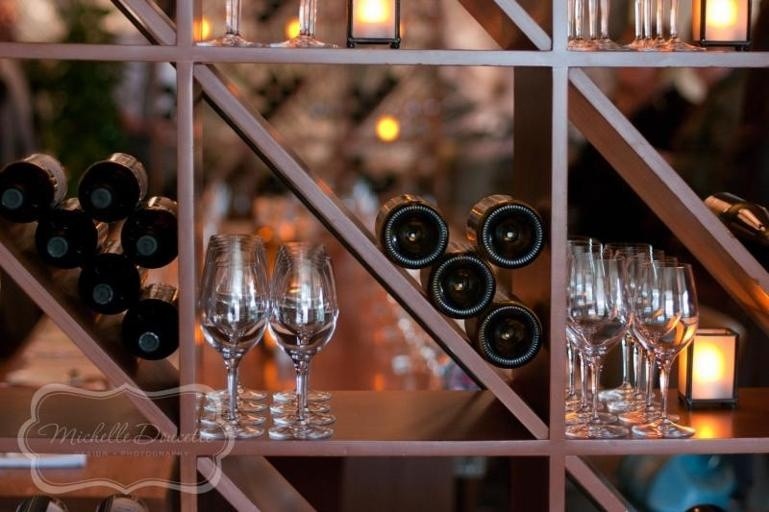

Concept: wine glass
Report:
left=564, top=239, right=701, bottom=439
left=196, top=234, right=337, bottom=440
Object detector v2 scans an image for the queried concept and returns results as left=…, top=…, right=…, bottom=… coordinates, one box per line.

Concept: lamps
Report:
left=676, top=326, right=741, bottom=410
left=344, top=1, right=403, bottom=51
left=690, top=0, right=754, bottom=52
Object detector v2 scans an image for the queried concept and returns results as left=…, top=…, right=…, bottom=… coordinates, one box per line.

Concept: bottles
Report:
left=703, top=191, right=768, bottom=248
left=374, top=192, right=544, bottom=370
left=2, top=152, right=179, bottom=360
